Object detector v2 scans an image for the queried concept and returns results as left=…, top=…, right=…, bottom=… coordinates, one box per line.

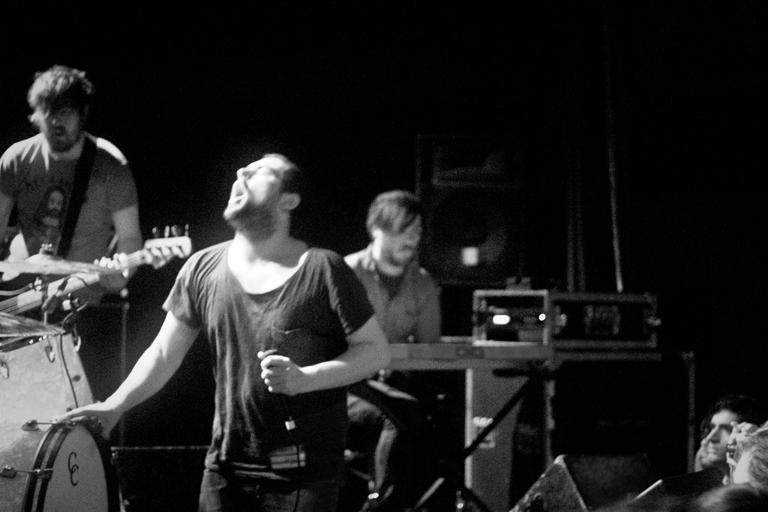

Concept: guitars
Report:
left=0, top=225, right=193, bottom=315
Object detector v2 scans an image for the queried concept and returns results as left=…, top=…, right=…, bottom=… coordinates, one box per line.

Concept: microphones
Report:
left=261, top=344, right=297, bottom=431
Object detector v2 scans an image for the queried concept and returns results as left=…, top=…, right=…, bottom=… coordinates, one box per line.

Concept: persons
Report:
left=55, top=154, right=392, bottom=510
left=603, top=397, right=767, bottom=512
left=338, top=190, right=444, bottom=502
left=0, top=64, right=144, bottom=391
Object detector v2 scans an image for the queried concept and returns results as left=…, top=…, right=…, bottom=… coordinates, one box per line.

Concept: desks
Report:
left=375, top=342, right=699, bottom=511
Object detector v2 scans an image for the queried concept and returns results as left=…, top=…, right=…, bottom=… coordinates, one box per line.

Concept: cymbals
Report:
left=0, top=310, right=67, bottom=337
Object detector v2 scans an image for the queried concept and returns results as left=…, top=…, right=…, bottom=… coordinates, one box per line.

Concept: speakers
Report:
left=17, top=303, right=130, bottom=445
left=417, top=135, right=527, bottom=286
left=509, top=454, right=671, bottom=512
left=465, top=351, right=695, bottom=512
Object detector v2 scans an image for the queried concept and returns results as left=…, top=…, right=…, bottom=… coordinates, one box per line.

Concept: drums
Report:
left=0, top=415, right=119, bottom=512
